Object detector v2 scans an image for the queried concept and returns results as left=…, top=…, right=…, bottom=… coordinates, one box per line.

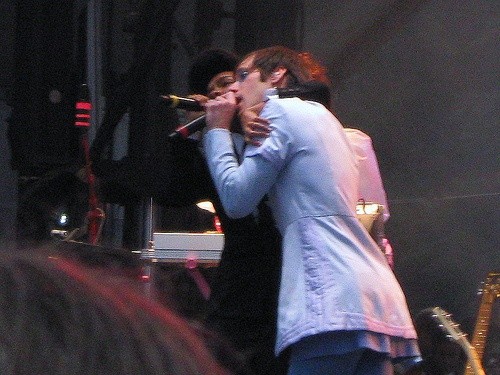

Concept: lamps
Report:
left=196, top=201, right=222, bottom=232
left=73, top=87, right=92, bottom=127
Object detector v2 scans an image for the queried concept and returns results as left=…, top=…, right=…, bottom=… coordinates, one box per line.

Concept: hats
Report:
left=190, top=49, right=240, bottom=95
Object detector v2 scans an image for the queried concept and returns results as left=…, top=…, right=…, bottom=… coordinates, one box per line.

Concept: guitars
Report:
left=464, top=273, right=500, bottom=375
left=431, top=307, right=486, bottom=375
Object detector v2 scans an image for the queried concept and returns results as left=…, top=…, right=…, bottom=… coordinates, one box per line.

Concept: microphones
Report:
left=159, top=94, right=205, bottom=111
left=167, top=114, right=207, bottom=142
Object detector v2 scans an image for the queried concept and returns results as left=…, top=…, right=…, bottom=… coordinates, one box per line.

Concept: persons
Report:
left=185, top=46, right=423, bottom=375
left=0, top=246, right=228, bottom=375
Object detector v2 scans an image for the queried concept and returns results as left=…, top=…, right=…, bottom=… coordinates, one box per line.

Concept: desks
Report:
left=152, top=232, right=225, bottom=250
